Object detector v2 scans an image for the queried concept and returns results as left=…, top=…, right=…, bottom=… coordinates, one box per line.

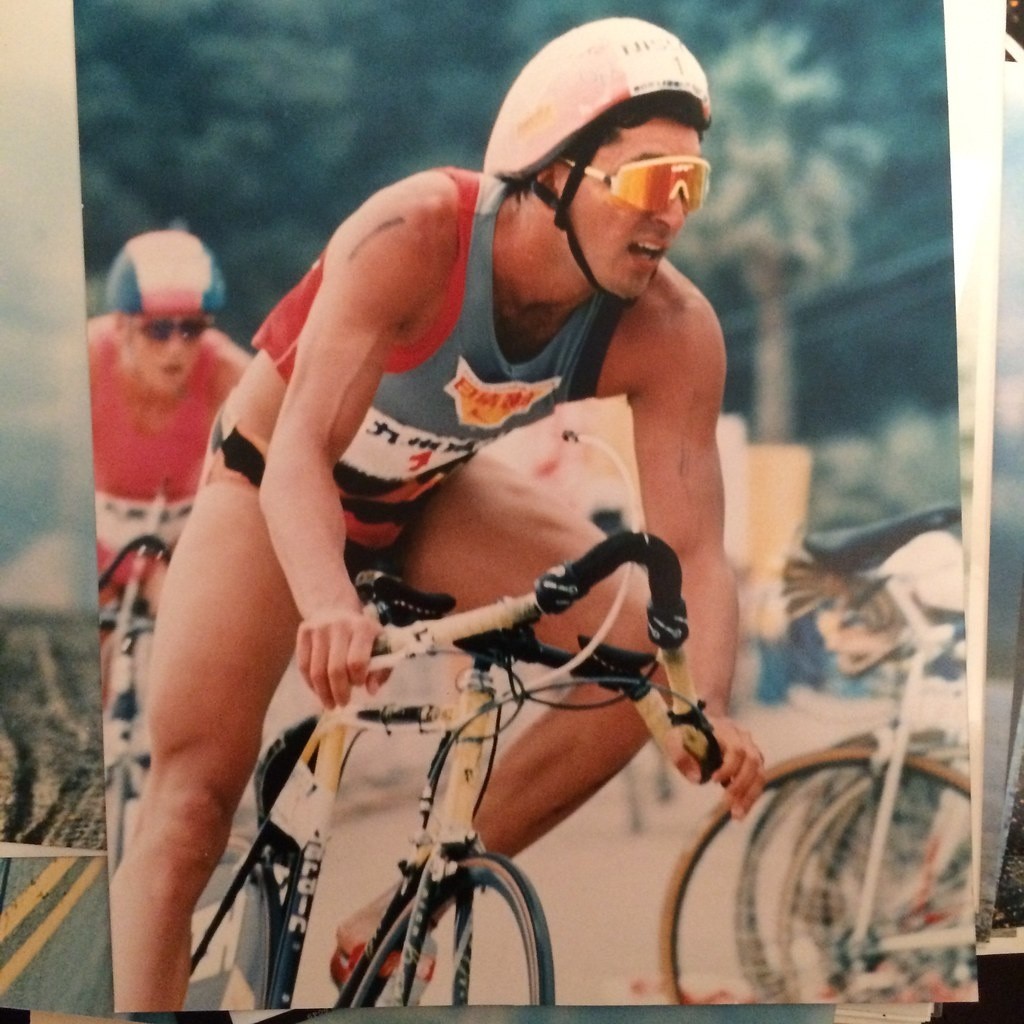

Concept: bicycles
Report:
left=107, top=487, right=984, bottom=1008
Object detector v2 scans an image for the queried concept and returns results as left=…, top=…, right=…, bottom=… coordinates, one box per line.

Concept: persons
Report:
left=83, top=225, right=261, bottom=708
left=114, top=17, right=763, bottom=1008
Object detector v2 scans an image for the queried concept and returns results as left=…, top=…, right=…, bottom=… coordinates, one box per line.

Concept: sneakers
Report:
left=330, top=935, right=438, bottom=1008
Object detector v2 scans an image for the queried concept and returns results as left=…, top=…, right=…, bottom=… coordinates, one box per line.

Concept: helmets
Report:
left=486, top=15, right=715, bottom=180
left=104, top=229, right=227, bottom=318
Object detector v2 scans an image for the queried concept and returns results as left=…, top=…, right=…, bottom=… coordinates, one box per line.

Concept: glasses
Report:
left=131, top=320, right=215, bottom=343
left=556, top=152, right=712, bottom=213
